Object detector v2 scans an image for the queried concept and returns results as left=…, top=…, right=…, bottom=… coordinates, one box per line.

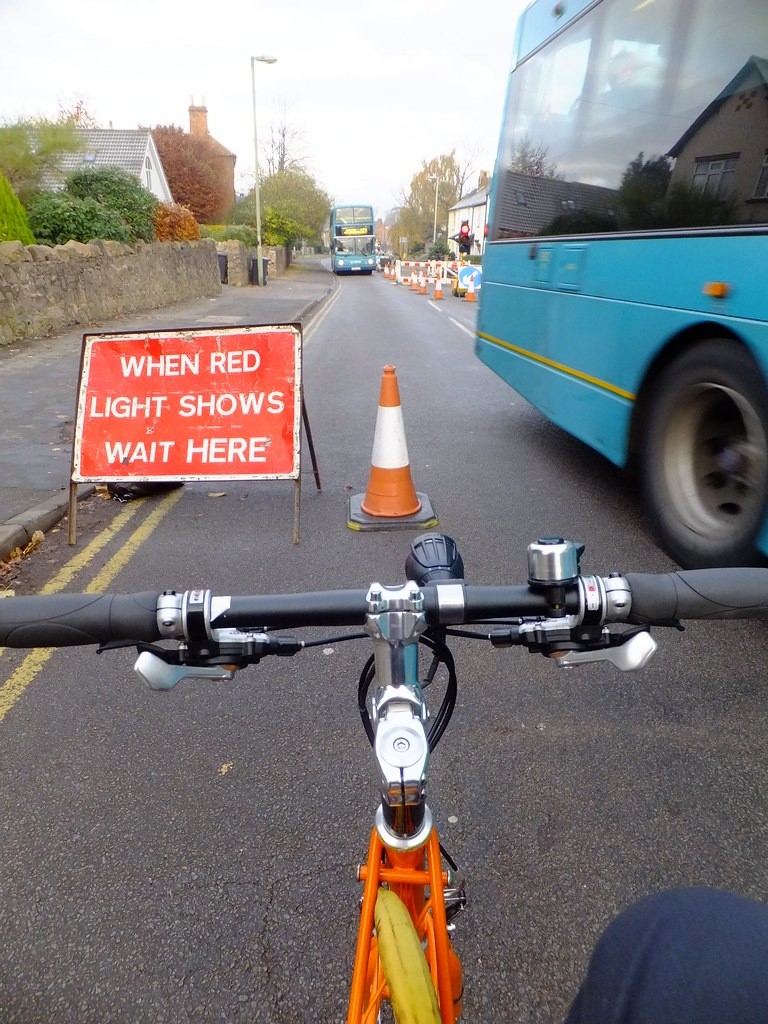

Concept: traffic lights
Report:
left=458, top=222, right=470, bottom=253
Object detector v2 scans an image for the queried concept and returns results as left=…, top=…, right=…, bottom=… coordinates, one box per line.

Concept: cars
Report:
left=376, top=255, right=394, bottom=272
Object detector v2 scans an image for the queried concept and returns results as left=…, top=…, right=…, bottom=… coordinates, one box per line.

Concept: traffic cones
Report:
left=462, top=275, right=478, bottom=302
left=344, top=364, right=441, bottom=532
left=383, top=263, right=419, bottom=291
left=429, top=273, right=447, bottom=301
left=413, top=272, right=430, bottom=296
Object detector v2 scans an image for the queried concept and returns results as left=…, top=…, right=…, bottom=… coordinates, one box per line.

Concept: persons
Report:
left=562, top=886, right=768, bottom=1024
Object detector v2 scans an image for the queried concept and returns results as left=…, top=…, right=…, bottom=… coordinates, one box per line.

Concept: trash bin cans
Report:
left=251, top=256, right=272, bottom=286
left=216, top=253, right=228, bottom=284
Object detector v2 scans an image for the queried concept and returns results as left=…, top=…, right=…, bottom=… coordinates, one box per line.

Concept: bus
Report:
left=472, top=0, right=768, bottom=574
left=329, top=204, right=376, bottom=276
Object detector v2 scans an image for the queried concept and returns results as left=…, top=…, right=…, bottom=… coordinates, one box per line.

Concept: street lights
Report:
left=249, top=54, right=278, bottom=285
left=428, top=175, right=439, bottom=246
